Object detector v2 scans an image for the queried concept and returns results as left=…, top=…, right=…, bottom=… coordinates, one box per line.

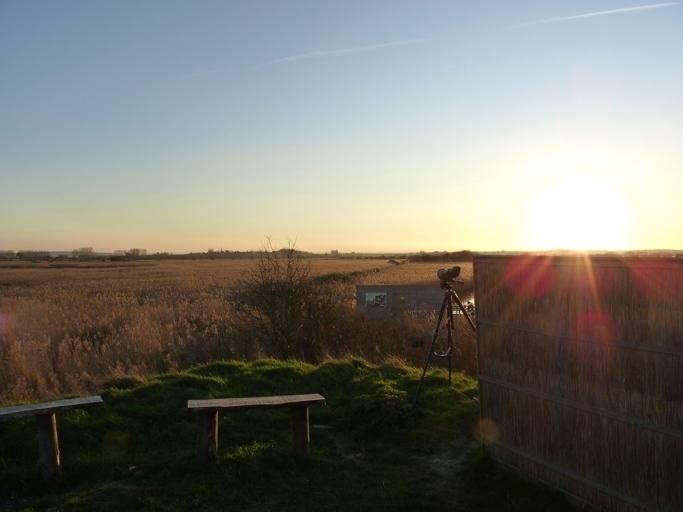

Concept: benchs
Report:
left=452, top=305, right=466, bottom=315
left=0, top=395, right=103, bottom=467
left=188, top=392, right=326, bottom=452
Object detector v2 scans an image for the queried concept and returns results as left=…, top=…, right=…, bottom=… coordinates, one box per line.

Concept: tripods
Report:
left=418, top=288, right=476, bottom=391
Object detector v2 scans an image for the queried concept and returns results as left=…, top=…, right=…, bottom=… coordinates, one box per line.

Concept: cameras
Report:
left=435, top=265, right=464, bottom=288
